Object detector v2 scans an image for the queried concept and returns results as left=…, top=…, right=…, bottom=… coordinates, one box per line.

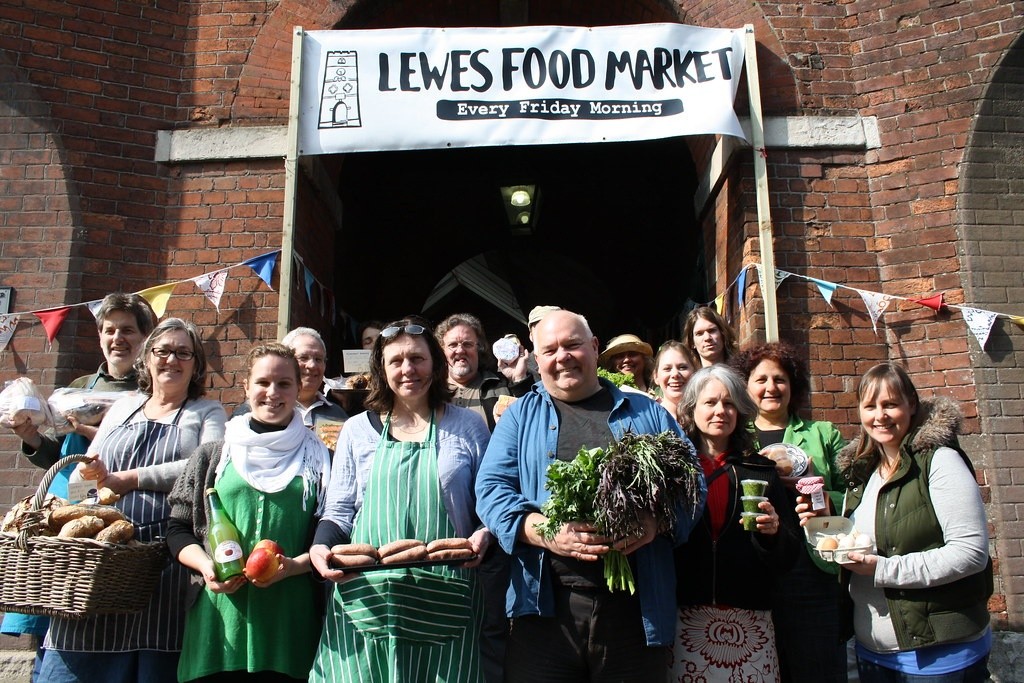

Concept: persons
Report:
left=227, top=327, right=349, bottom=454
left=795, top=363, right=994, bottom=683
left=474, top=310, right=707, bottom=683
left=37, top=317, right=228, bottom=683
left=683, top=306, right=739, bottom=370
left=676, top=364, right=808, bottom=683
left=652, top=339, right=699, bottom=422
left=435, top=312, right=534, bottom=437
left=527, top=305, right=561, bottom=372
left=166, top=343, right=331, bottom=683
left=331, top=316, right=386, bottom=417
left=596, top=333, right=653, bottom=392
left=308, top=317, right=491, bottom=683
left=0, top=294, right=159, bottom=683
left=732, top=341, right=848, bottom=683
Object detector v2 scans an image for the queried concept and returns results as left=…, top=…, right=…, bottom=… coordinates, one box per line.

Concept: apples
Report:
left=246, top=539, right=284, bottom=583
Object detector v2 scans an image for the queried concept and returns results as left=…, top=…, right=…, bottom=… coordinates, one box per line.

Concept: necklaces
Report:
left=391, top=415, right=429, bottom=434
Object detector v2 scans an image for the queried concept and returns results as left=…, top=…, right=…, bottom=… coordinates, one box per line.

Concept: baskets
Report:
left=0, top=454, right=170, bottom=620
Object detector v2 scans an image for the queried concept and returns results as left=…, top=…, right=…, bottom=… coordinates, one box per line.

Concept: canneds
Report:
left=796, top=477, right=826, bottom=513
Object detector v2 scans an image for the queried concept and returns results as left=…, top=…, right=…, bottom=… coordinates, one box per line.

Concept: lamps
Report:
left=516, top=212, right=530, bottom=223
left=510, top=189, right=532, bottom=206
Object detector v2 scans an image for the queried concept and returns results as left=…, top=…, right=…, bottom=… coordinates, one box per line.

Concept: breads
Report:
left=329, top=537, right=472, bottom=566
left=492, top=337, right=519, bottom=364
left=343, top=373, right=373, bottom=389
left=0, top=377, right=112, bottom=435
left=0, top=487, right=135, bottom=545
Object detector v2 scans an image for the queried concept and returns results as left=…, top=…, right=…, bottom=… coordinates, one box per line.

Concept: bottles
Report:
left=205, top=488, right=245, bottom=582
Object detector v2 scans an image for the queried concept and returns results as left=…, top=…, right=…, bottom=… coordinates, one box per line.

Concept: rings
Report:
left=520, top=352, right=524, bottom=357
left=861, top=554, right=865, bottom=562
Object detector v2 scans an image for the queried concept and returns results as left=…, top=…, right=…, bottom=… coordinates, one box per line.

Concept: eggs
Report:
left=816, top=534, right=872, bottom=552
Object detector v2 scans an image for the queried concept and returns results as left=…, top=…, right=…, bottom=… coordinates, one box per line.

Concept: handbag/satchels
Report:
left=658, top=600, right=781, bottom=683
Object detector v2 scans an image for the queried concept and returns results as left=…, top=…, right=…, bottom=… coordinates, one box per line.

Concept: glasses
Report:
left=147, top=348, right=197, bottom=361
left=379, top=325, right=427, bottom=339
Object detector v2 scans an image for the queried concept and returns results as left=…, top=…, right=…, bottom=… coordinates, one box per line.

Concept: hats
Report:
left=598, top=334, right=653, bottom=371
left=527, top=305, right=561, bottom=331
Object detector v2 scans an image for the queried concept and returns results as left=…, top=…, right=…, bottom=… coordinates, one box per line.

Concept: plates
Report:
left=758, top=442, right=808, bottom=477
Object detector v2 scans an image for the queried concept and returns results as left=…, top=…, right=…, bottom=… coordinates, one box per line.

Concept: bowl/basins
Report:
left=742, top=479, right=768, bottom=531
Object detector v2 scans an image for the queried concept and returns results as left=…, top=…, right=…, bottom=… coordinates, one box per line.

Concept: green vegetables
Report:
left=530, top=419, right=703, bottom=596
left=598, top=367, right=639, bottom=390
